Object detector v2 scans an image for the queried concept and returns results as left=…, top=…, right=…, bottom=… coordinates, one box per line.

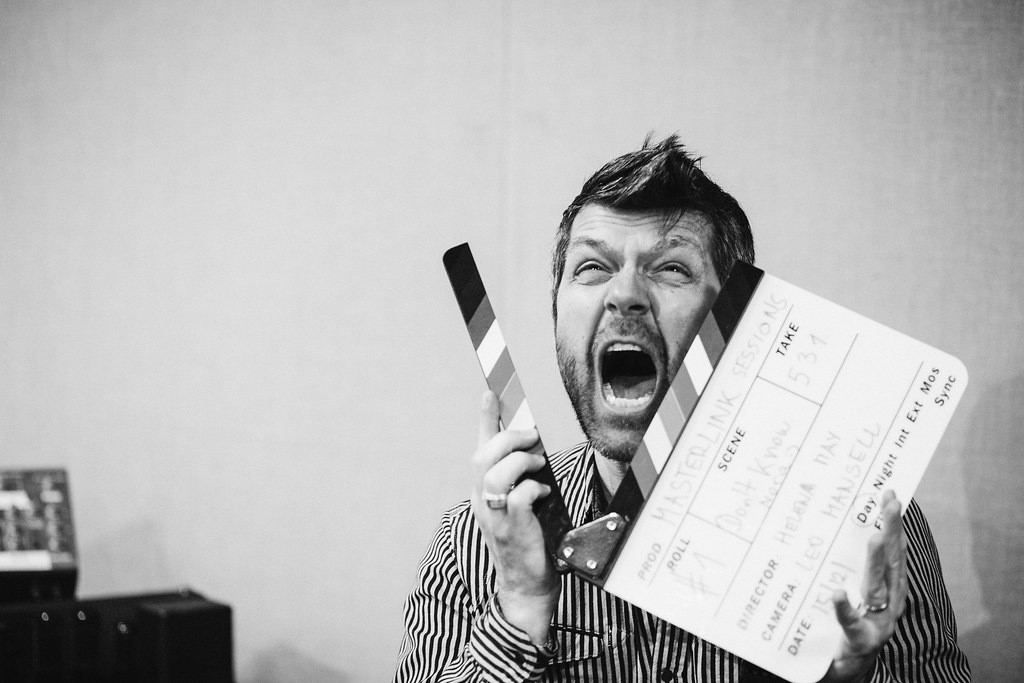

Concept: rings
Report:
left=865, top=603, right=887, bottom=612
left=484, top=494, right=507, bottom=508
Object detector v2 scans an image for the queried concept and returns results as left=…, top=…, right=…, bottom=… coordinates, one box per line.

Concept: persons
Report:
left=393, top=133, right=970, bottom=683
